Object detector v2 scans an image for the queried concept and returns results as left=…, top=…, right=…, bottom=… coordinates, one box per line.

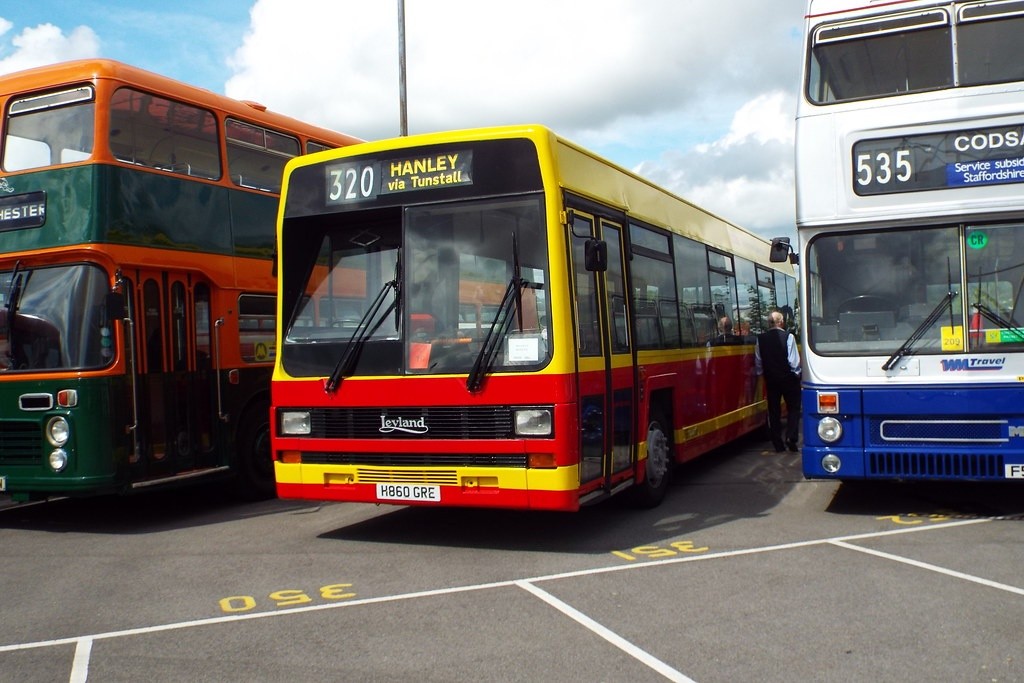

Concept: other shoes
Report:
left=774, top=440, right=785, bottom=452
left=785, top=438, right=798, bottom=452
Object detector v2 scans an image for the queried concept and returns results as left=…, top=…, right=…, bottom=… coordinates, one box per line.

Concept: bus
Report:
left=767, top=0, right=1024, bottom=483
left=271, top=124, right=824, bottom=512
left=0, top=59, right=540, bottom=495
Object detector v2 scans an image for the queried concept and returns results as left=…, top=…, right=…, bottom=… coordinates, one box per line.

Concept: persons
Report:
left=705, top=317, right=753, bottom=347
left=755, top=312, right=802, bottom=452
